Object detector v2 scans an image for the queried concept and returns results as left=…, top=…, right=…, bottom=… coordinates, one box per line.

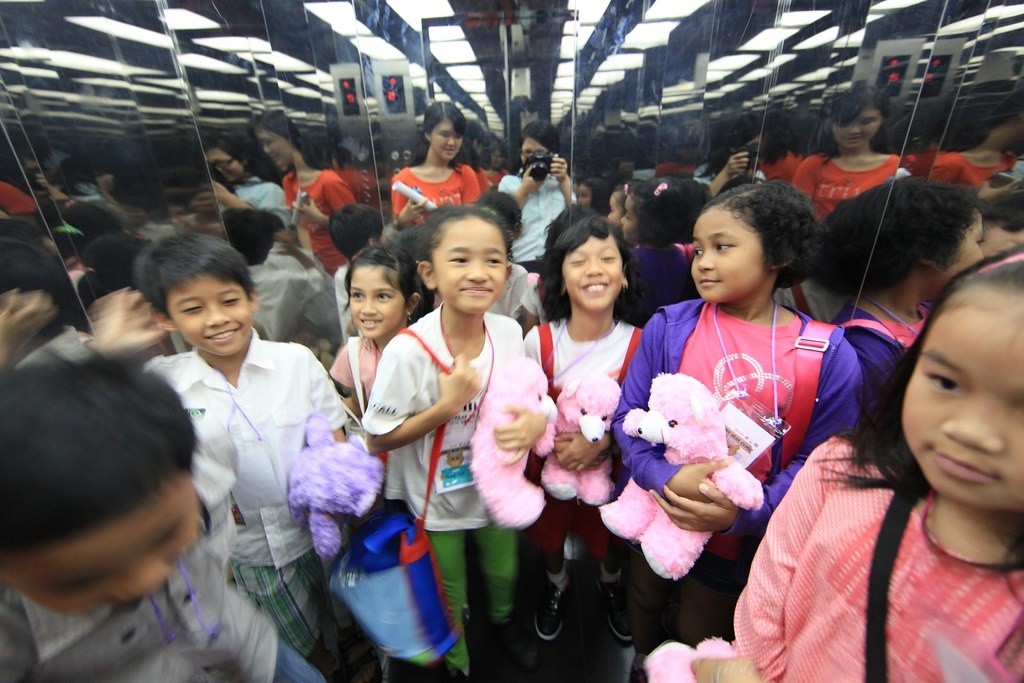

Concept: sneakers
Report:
left=594, top=573, right=632, bottom=641
left=534, top=575, right=575, bottom=641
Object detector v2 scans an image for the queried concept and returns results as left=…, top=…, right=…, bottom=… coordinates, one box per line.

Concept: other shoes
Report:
left=488, top=617, right=540, bottom=672
left=659, top=597, right=680, bottom=640
left=629, top=653, right=648, bottom=683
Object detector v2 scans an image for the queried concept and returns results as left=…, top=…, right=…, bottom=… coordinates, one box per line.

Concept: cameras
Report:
left=20, top=166, right=49, bottom=193
left=738, top=142, right=763, bottom=171
left=525, top=149, right=558, bottom=182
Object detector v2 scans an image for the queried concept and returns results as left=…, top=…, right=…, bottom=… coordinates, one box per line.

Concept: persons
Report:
left=0, top=103, right=1024, bottom=683
left=0, top=78, right=1024, bottom=418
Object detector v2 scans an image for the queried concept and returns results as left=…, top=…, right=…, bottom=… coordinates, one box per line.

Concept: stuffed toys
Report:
left=287, top=412, right=384, bottom=558
left=599, top=372, right=763, bottom=581
left=641, top=636, right=738, bottom=683
left=538, top=368, right=622, bottom=505
left=468, top=355, right=558, bottom=530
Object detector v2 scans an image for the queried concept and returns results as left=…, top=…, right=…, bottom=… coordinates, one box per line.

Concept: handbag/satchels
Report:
left=323, top=508, right=463, bottom=668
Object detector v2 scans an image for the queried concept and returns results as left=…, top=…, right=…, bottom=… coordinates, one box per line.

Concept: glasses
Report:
left=520, top=148, right=550, bottom=158
left=208, top=158, right=236, bottom=169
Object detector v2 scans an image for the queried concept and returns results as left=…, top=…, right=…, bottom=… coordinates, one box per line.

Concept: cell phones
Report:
left=990, top=173, right=1014, bottom=189
left=300, top=192, right=311, bottom=207
